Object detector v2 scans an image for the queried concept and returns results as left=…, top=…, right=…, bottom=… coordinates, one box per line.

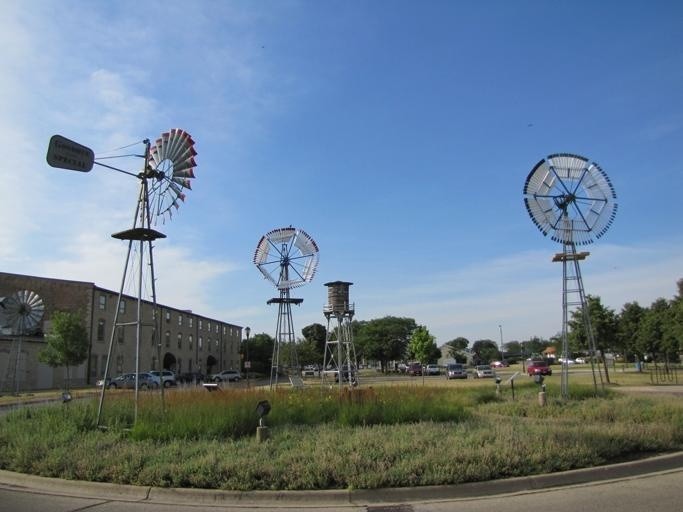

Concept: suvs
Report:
left=445, top=364, right=468, bottom=380
left=335, top=365, right=356, bottom=382
left=207, top=370, right=242, bottom=382
left=147, top=370, right=176, bottom=388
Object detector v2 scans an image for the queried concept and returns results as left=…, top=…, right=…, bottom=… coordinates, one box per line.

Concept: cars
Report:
left=180, top=372, right=204, bottom=383
left=557, top=357, right=587, bottom=365
left=397, top=360, right=440, bottom=376
left=527, top=360, right=552, bottom=377
left=473, top=364, right=496, bottom=379
left=95, top=373, right=161, bottom=390
